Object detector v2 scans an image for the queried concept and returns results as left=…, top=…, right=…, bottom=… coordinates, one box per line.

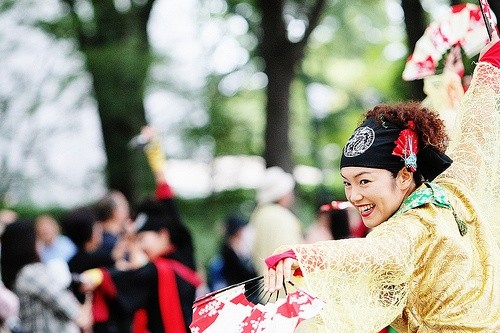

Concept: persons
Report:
left=308, top=42, right=465, bottom=240
left=263, top=30, right=500, bottom=333
left=0, top=121, right=200, bottom=333
left=247, top=166, right=305, bottom=305
left=206, top=214, right=260, bottom=306
